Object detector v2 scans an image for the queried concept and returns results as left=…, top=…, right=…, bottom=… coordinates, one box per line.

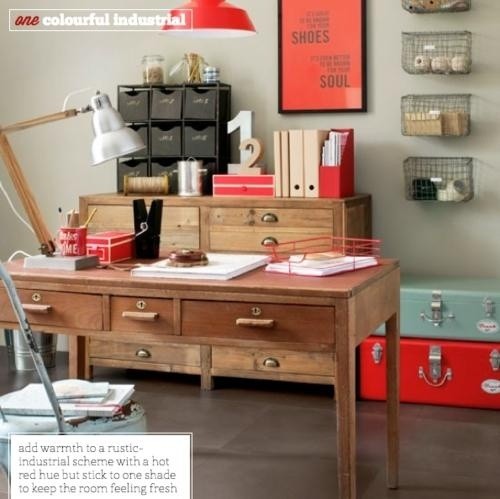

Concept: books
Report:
left=130, top=251, right=270, bottom=281
left=265, top=251, right=378, bottom=277
left=0, top=379, right=135, bottom=418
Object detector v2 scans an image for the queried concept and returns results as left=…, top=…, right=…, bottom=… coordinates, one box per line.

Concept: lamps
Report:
left=0, top=89, right=147, bottom=272
left=161, top=0, right=258, bottom=33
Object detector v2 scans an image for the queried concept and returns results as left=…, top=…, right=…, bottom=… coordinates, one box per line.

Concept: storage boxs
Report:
left=359, top=276, right=499, bottom=412
left=86, top=230, right=136, bottom=264
left=117, top=84, right=232, bottom=195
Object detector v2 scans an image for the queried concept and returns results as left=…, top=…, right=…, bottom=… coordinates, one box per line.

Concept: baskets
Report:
left=400, top=1, right=473, bottom=204
left=263, top=235, right=380, bottom=278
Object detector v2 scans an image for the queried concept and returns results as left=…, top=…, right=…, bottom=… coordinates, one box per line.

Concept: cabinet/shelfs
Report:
left=69, top=191, right=372, bottom=401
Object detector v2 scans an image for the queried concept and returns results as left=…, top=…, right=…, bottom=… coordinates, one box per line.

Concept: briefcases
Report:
left=359, top=277, right=500, bottom=410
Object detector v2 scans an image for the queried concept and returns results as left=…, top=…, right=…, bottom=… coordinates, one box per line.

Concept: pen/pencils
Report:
left=58, top=207, right=75, bottom=228
left=84, top=208, right=98, bottom=227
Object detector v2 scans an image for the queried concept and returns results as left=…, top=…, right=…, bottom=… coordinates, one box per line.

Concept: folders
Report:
left=289, top=128, right=329, bottom=198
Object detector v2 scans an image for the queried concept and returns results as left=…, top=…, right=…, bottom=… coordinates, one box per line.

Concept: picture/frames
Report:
left=277, top=0, right=368, bottom=114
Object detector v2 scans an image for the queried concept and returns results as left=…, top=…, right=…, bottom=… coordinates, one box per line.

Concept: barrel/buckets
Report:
left=11, top=330, right=57, bottom=372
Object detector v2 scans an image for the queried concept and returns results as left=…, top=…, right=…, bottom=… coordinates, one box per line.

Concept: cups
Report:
left=176, top=159, right=204, bottom=198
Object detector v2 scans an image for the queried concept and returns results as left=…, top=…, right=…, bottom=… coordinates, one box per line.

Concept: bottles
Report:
left=141, top=53, right=166, bottom=87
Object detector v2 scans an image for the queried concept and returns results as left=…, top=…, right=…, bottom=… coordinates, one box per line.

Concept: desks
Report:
left=0, top=247, right=400, bottom=498
